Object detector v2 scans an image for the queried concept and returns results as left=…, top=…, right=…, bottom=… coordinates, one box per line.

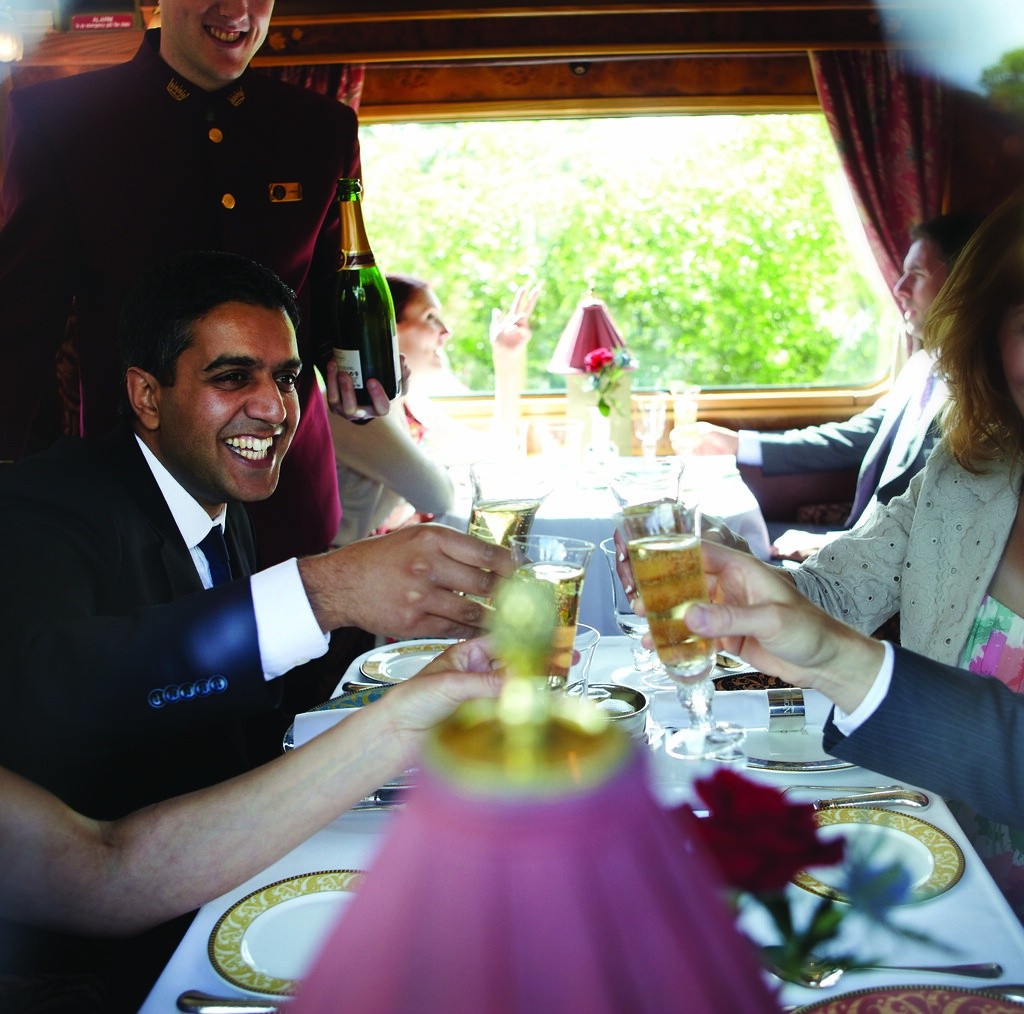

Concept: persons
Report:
left=0, top=0, right=410, bottom=716
left=0, top=275, right=582, bottom=1014
left=611, top=194, right=1024, bottom=914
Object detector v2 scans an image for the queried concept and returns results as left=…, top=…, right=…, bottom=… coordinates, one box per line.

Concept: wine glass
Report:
left=633, top=401, right=665, bottom=466
left=448, top=461, right=747, bottom=760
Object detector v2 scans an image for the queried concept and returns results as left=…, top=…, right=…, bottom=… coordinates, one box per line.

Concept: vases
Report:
left=579, top=414, right=617, bottom=484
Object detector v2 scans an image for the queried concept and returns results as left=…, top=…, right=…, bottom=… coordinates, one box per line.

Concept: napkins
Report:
left=647, top=688, right=835, bottom=732
left=293, top=706, right=366, bottom=749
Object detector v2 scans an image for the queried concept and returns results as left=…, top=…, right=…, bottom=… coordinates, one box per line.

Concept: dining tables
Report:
left=139, top=631, right=1024, bottom=1014
left=449, top=455, right=772, bottom=637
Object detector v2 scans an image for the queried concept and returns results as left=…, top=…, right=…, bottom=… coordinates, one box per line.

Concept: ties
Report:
left=920, top=375, right=935, bottom=411
left=197, top=524, right=232, bottom=587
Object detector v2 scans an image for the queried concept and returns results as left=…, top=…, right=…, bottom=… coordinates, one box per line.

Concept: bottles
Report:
left=322, top=177, right=401, bottom=407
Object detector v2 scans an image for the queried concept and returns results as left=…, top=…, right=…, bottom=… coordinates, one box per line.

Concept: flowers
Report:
left=574, top=345, right=639, bottom=416
left=658, top=763, right=973, bottom=1004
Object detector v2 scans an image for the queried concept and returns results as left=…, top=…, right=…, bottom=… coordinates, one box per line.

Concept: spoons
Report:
left=760, top=945, right=1005, bottom=990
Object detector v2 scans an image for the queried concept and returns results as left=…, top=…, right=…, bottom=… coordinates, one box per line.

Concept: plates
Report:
left=787, top=805, right=966, bottom=910
left=741, top=726, right=858, bottom=773
left=358, top=637, right=466, bottom=684
left=207, top=868, right=369, bottom=1000
left=283, top=681, right=395, bottom=752
left=788, top=984, right=1023, bottom=1014
